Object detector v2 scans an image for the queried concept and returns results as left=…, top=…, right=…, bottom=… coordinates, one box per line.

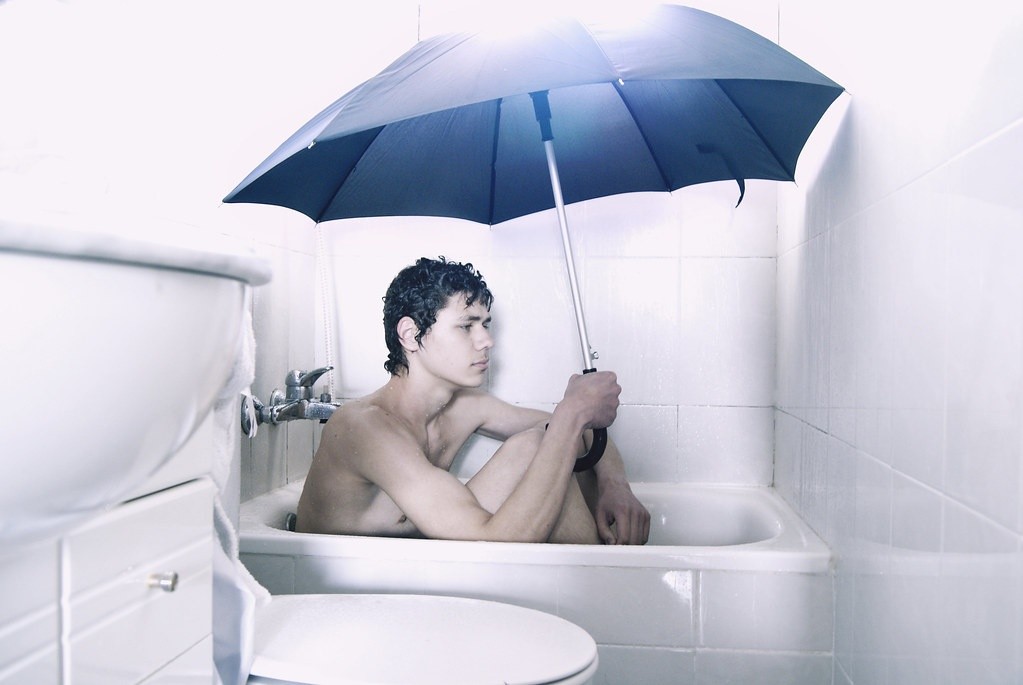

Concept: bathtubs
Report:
left=235, top=473, right=838, bottom=685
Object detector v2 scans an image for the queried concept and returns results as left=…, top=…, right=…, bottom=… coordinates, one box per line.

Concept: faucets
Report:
left=258, top=366, right=341, bottom=437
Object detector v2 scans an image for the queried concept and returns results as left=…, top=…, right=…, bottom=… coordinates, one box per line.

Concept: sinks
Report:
left=1, top=219, right=276, bottom=550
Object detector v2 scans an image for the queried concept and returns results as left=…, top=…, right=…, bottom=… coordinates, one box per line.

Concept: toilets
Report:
left=243, top=594, right=601, bottom=684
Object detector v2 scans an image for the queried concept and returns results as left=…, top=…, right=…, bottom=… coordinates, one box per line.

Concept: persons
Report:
left=286, top=258, right=651, bottom=545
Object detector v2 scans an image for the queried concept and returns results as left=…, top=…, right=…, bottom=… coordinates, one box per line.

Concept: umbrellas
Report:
left=219, top=0, right=847, bottom=472
